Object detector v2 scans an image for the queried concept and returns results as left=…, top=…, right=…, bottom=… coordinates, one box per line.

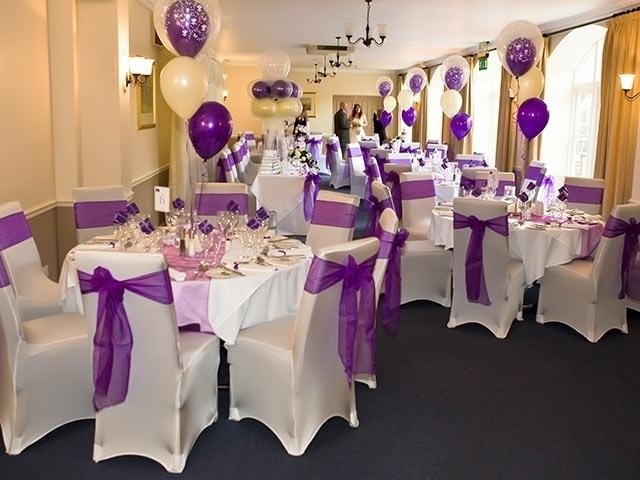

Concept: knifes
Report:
left=269, top=236, right=298, bottom=243
left=223, top=266, right=246, bottom=277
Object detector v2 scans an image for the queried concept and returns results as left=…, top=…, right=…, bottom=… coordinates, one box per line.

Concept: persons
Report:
left=372, top=108, right=387, bottom=146
left=349, top=104, right=369, bottom=143
left=333, top=100, right=352, bottom=155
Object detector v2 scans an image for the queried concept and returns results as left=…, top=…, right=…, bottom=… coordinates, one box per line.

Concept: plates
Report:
left=204, top=268, right=231, bottom=279
left=269, top=254, right=306, bottom=265
left=566, top=207, right=599, bottom=225
left=527, top=225, right=543, bottom=229
left=274, top=239, right=300, bottom=248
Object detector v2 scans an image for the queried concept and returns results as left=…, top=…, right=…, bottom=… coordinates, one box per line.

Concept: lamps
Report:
left=305, top=64, right=325, bottom=87
left=121, top=55, right=156, bottom=92
left=618, top=71, right=640, bottom=101
left=325, top=37, right=353, bottom=70
left=314, top=54, right=339, bottom=78
left=342, top=0, right=392, bottom=47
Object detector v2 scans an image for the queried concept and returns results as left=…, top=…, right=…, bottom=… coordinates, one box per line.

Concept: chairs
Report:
left=1, top=260, right=104, bottom=458
left=73, top=183, right=136, bottom=246
left=65, top=246, right=222, bottom=475
left=1, top=199, right=64, bottom=314
left=354, top=209, right=406, bottom=394
left=224, top=232, right=381, bottom=455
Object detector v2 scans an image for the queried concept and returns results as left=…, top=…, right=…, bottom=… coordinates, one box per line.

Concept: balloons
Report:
left=376, top=54, right=473, bottom=141
left=517, top=96, right=550, bottom=143
left=150, top=1, right=224, bottom=57
left=246, top=45, right=304, bottom=141
left=497, top=19, right=544, bottom=79
left=512, top=67, right=545, bottom=104
left=188, top=101, right=233, bottom=161
left=158, top=55, right=209, bottom=125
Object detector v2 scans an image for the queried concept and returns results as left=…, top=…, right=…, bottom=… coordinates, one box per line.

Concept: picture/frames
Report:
left=296, top=90, right=317, bottom=120
left=134, top=60, right=157, bottom=131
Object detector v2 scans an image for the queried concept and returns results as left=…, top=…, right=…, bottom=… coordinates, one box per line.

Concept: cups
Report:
left=463, top=183, right=497, bottom=200
left=266, top=210, right=277, bottom=234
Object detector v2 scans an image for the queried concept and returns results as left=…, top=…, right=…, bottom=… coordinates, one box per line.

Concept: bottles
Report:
left=501, top=185, right=521, bottom=203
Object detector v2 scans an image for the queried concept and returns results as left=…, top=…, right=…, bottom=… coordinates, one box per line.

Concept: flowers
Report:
left=109, top=198, right=274, bottom=235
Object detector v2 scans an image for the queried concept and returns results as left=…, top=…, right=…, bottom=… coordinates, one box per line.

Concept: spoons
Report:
left=256, top=256, right=279, bottom=271
left=194, top=264, right=209, bottom=281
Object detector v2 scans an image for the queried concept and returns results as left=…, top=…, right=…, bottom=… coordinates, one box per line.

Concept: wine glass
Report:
left=518, top=198, right=569, bottom=224
left=111, top=206, right=267, bottom=267
